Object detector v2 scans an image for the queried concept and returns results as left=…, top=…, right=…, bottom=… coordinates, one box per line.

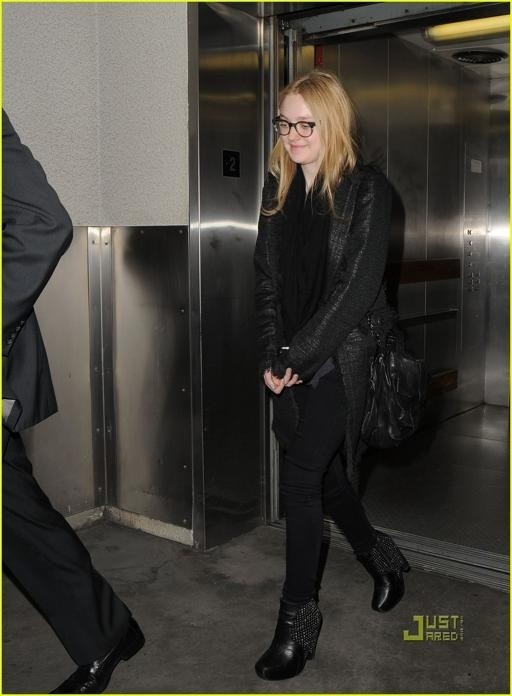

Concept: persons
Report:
left=2, top=106, right=145, bottom=694
left=252, top=69, right=411, bottom=681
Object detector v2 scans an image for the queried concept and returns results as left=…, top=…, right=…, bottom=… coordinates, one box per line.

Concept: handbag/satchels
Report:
left=361, top=339, right=425, bottom=449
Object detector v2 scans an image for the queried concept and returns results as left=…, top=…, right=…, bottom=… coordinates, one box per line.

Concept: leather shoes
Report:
left=49, top=615, right=145, bottom=694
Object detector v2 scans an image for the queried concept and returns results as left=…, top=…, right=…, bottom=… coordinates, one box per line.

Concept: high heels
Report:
left=255, top=597, right=323, bottom=681
left=356, top=530, right=411, bottom=613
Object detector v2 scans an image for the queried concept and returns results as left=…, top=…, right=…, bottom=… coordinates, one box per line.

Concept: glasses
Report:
left=272, top=116, right=321, bottom=138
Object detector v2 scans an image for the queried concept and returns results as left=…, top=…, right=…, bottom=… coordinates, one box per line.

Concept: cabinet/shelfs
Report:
left=323, top=31, right=494, bottom=432
